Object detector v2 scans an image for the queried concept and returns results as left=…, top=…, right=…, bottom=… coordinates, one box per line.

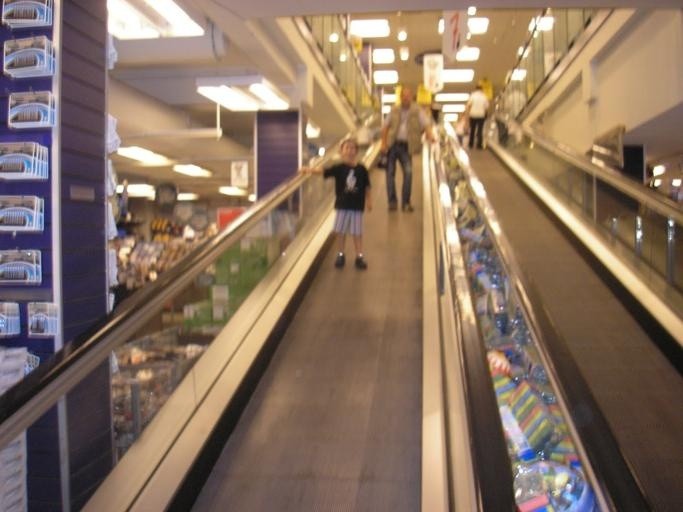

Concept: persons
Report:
left=378, top=82, right=436, bottom=213
left=297, top=138, right=372, bottom=270
left=460, top=80, right=492, bottom=151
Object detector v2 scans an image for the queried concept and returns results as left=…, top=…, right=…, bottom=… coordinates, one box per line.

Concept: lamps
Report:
left=190, top=73, right=291, bottom=114
left=106, top=0, right=211, bottom=42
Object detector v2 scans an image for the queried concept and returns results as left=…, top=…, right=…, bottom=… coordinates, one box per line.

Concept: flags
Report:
left=442, top=10, right=469, bottom=63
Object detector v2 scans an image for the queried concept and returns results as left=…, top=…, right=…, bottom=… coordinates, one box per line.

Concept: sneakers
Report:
left=355, top=257, right=368, bottom=270
left=388, top=203, right=413, bottom=213
left=334, top=252, right=346, bottom=268
